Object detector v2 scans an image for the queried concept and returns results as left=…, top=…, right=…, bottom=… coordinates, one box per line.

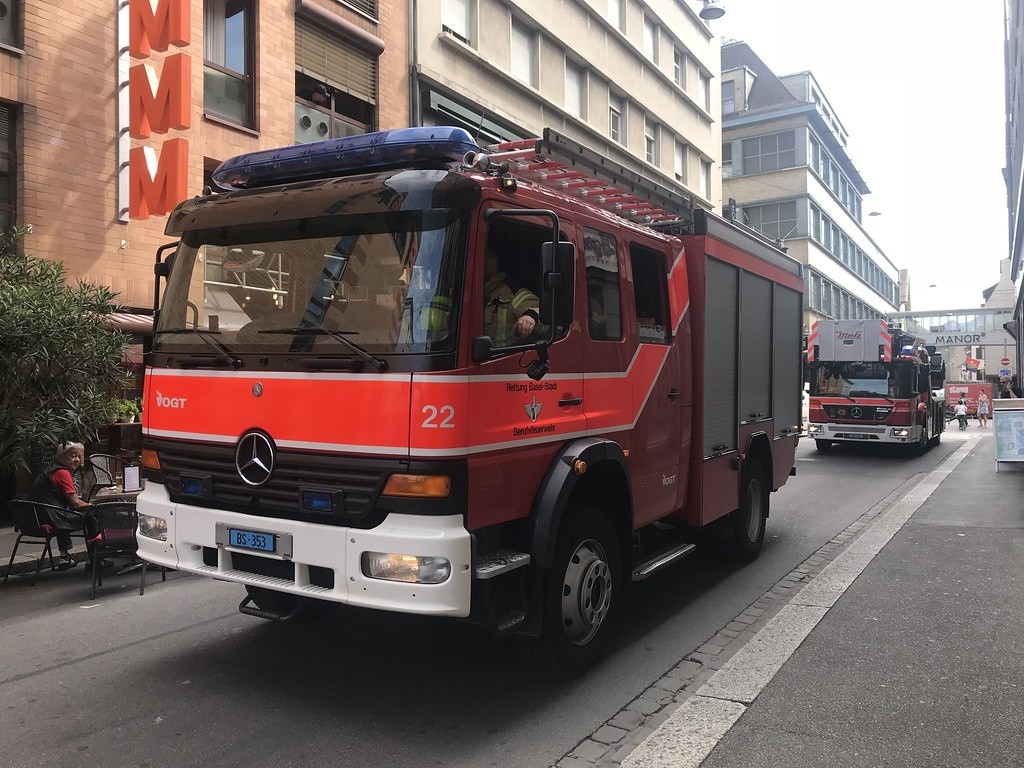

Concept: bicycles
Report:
left=958, top=417, right=966, bottom=430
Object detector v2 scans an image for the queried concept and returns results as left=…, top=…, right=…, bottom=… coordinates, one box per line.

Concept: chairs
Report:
left=3, top=499, right=103, bottom=587
left=86, top=502, right=166, bottom=600
left=87, top=453, right=133, bottom=503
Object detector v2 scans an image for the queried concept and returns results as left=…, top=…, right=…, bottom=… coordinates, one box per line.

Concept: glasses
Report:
left=999, top=382, right=1005, bottom=384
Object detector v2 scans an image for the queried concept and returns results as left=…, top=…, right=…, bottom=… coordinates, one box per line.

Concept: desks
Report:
left=95, top=484, right=143, bottom=503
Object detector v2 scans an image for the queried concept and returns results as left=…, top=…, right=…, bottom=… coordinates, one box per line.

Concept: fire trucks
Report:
left=134, top=123, right=806, bottom=662
left=806, top=319, right=946, bottom=457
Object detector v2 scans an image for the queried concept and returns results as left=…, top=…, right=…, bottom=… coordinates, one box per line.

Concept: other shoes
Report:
left=977, top=425, right=987, bottom=428
left=959, top=425, right=966, bottom=430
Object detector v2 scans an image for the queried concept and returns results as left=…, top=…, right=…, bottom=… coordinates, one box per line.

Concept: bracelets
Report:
left=89, top=502, right=91, bottom=506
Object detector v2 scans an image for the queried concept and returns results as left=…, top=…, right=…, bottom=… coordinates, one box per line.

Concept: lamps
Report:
left=699, top=0, right=727, bottom=20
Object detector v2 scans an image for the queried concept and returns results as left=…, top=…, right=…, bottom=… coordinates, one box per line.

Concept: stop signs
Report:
left=1001, top=358, right=1010, bottom=366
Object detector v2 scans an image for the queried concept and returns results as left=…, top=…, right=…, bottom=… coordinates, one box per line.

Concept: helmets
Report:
left=416, top=295, right=453, bottom=332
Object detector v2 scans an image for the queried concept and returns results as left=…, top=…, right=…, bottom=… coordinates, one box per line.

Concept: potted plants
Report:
left=99, top=396, right=143, bottom=423
left=0, top=224, right=138, bottom=529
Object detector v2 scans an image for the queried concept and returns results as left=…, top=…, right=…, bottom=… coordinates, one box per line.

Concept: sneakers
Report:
left=86, top=558, right=115, bottom=569
left=58, top=558, right=78, bottom=571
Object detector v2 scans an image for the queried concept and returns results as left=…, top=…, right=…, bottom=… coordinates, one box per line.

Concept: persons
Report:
left=977, top=389, right=989, bottom=428
left=28, top=441, right=115, bottom=570
left=954, top=393, right=968, bottom=427
left=1000, top=376, right=1022, bottom=398
left=448, top=249, right=540, bottom=348
left=298, top=84, right=330, bottom=104
